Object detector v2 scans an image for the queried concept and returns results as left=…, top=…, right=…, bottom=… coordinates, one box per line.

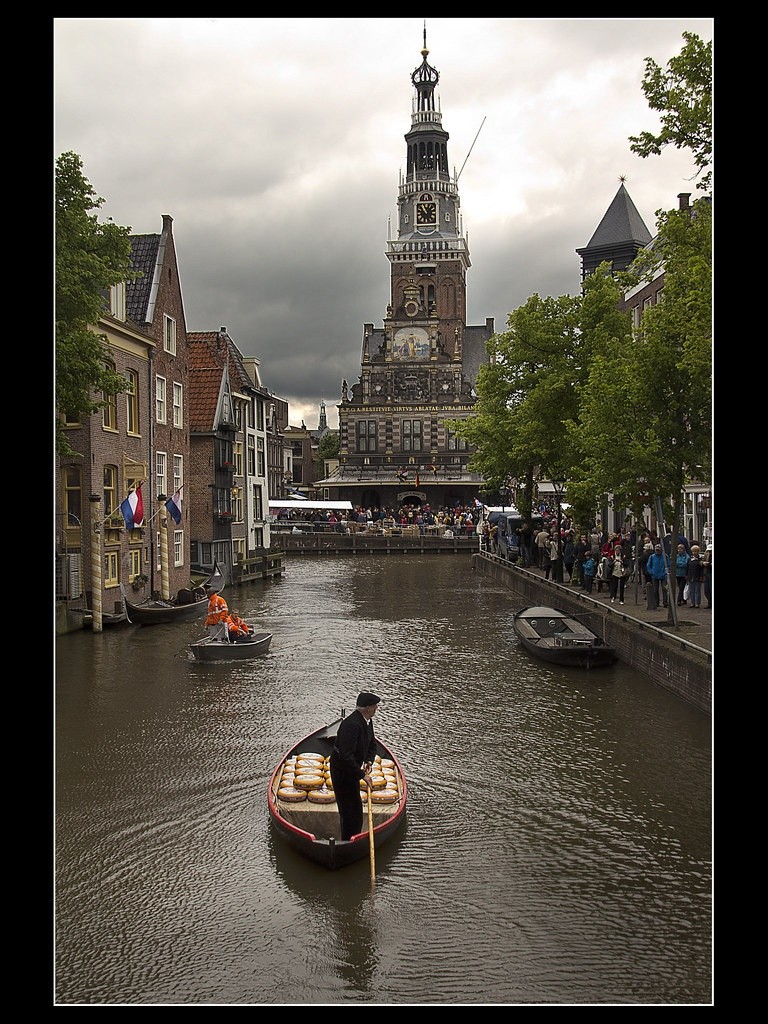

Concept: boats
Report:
left=266, top=701, right=406, bottom=865
left=188, top=633, right=274, bottom=660
left=123, top=553, right=227, bottom=625
left=511, top=604, right=616, bottom=667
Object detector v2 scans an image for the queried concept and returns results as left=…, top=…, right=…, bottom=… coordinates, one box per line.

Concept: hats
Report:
left=357, top=693, right=380, bottom=706
left=706, top=544, right=712, bottom=551
left=655, top=544, right=662, bottom=551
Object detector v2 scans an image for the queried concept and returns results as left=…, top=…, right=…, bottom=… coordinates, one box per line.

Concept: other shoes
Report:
left=619, top=601, right=624, bottom=605
left=704, top=605, right=710, bottom=609
left=610, top=599, right=615, bottom=603
left=688, top=605, right=699, bottom=608
left=677, top=601, right=682, bottom=606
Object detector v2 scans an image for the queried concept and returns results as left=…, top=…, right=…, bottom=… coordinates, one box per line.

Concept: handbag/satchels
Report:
left=683, top=582, right=690, bottom=600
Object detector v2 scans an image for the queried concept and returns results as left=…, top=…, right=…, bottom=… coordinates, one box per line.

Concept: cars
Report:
left=496, top=511, right=544, bottom=562
left=477, top=506, right=522, bottom=557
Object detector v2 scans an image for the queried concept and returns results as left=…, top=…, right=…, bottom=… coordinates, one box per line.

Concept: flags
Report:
left=164, top=490, right=182, bottom=525
left=120, top=484, right=143, bottom=529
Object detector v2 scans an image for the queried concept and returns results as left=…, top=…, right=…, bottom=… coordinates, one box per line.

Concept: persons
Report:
left=270, top=497, right=712, bottom=610
left=204, top=588, right=248, bottom=641
left=328, top=693, right=380, bottom=842
